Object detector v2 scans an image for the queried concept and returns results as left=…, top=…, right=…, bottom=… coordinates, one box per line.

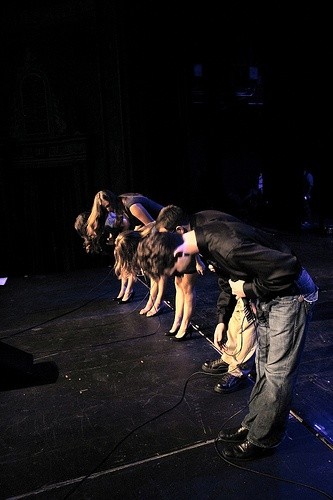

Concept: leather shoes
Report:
left=202, top=358, right=229, bottom=373
left=214, top=373, right=245, bottom=392
left=218, top=425, right=249, bottom=442
left=222, top=440, right=276, bottom=461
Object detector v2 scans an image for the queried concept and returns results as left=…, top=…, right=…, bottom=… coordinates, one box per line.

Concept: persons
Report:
left=155, top=204, right=255, bottom=394
left=74, top=190, right=207, bottom=343
left=138, top=221, right=319, bottom=460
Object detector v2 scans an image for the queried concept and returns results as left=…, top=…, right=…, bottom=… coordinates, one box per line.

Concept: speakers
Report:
left=0, top=340, right=34, bottom=392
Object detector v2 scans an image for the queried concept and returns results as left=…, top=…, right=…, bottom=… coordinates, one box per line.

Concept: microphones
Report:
left=230, top=274, right=250, bottom=311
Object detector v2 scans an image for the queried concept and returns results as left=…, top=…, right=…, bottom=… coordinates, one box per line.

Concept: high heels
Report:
left=169, top=324, right=192, bottom=342
left=146, top=307, right=161, bottom=317
left=139, top=306, right=153, bottom=314
left=113, top=294, right=124, bottom=301
left=164, top=325, right=180, bottom=336
left=118, top=290, right=134, bottom=304
